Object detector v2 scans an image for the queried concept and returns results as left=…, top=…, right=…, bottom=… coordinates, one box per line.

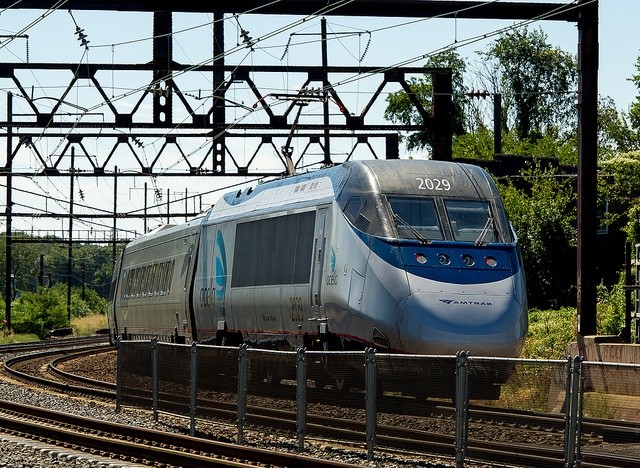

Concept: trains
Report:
left=106, top=158, right=529, bottom=401
